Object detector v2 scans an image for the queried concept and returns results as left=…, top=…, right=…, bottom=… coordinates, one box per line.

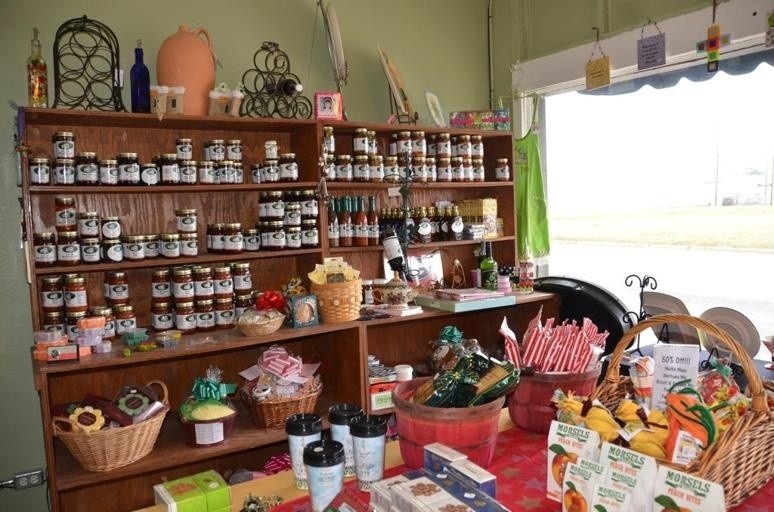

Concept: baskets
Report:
left=52, top=379, right=170, bottom=472
left=239, top=381, right=323, bottom=429
left=310, top=278, right=363, bottom=324
left=588, top=313, right=773, bottom=512
left=233, top=316, right=286, bottom=336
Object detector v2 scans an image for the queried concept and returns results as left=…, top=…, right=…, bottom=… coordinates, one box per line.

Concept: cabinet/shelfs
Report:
left=363, top=290, right=561, bottom=417
left=17, top=106, right=326, bottom=333
left=320, top=119, right=519, bottom=288
left=38, top=321, right=367, bottom=511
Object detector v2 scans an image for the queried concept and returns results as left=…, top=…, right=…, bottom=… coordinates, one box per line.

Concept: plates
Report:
left=640, top=292, right=699, bottom=345
left=697, top=307, right=760, bottom=363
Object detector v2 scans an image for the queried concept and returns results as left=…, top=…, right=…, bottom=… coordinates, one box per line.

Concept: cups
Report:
left=285, top=406, right=386, bottom=512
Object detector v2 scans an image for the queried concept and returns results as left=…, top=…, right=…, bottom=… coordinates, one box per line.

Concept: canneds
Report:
left=207, top=222, right=260, bottom=252
left=123, top=209, right=202, bottom=260
left=256, top=190, right=327, bottom=248
left=33, top=197, right=123, bottom=264
left=494, top=156, right=510, bottom=182
left=40, top=270, right=137, bottom=344
left=251, top=153, right=300, bottom=183
left=385, top=131, right=486, bottom=182
left=200, top=138, right=245, bottom=183
left=150, top=261, right=255, bottom=333
left=325, top=127, right=385, bottom=183
left=29, top=130, right=197, bottom=186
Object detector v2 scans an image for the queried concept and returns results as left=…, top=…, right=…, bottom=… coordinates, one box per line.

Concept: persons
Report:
left=321, top=97, right=335, bottom=115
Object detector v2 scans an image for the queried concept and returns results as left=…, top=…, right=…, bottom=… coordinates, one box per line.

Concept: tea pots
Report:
left=372, top=271, right=424, bottom=309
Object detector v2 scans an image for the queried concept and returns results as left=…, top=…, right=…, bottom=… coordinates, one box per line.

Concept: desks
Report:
left=129, top=406, right=773, bottom=512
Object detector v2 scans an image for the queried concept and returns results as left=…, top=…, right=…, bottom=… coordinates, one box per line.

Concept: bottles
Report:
left=27, top=39, right=49, bottom=108
left=519, top=246, right=534, bottom=294
left=130, top=47, right=150, bottom=113
left=479, top=241, right=498, bottom=291
left=328, top=195, right=379, bottom=248
left=379, top=228, right=412, bottom=282
left=380, top=206, right=464, bottom=244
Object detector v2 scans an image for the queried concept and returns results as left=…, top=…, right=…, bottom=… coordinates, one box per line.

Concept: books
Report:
left=431, top=287, right=505, bottom=301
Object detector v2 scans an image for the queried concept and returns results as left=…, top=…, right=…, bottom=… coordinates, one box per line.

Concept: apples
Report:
left=548, top=442, right=580, bottom=489
left=561, top=482, right=589, bottom=512
left=656, top=492, right=691, bottom=512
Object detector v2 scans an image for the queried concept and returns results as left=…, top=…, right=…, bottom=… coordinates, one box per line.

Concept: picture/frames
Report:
left=313, top=91, right=343, bottom=122
